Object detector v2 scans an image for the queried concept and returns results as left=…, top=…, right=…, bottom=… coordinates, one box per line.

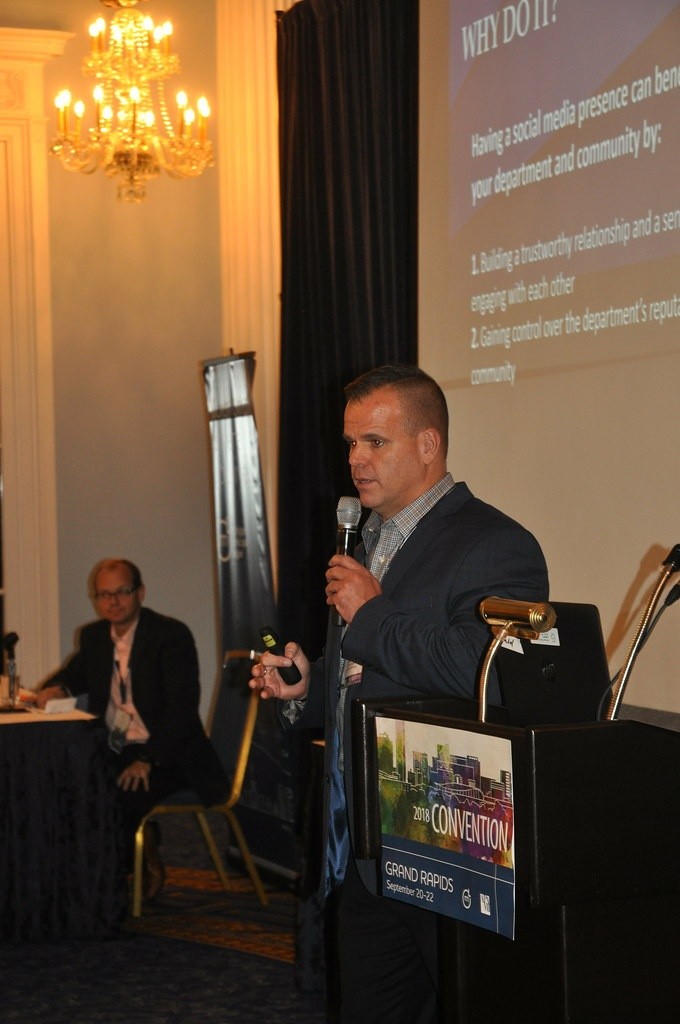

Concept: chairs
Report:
left=132, top=651, right=270, bottom=918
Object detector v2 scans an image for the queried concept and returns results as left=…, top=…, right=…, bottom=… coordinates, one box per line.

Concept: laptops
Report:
left=496, top=603, right=614, bottom=728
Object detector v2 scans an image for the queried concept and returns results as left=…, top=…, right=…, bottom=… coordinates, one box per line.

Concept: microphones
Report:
left=595, top=544, right=680, bottom=722
left=333, top=496, right=361, bottom=627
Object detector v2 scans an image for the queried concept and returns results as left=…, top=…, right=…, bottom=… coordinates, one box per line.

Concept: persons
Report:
left=247, top=365, right=550, bottom=1024
left=28, top=559, right=213, bottom=920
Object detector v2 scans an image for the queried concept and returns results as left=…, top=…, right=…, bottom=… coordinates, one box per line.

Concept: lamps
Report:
left=49, top=0, right=215, bottom=204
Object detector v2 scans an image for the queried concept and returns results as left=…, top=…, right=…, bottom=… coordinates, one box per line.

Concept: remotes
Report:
left=261, top=627, right=302, bottom=686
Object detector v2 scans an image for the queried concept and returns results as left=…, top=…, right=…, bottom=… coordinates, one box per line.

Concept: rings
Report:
left=262, top=665, right=268, bottom=676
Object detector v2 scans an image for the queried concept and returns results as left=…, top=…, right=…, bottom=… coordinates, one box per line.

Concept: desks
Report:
left=0, top=676, right=106, bottom=953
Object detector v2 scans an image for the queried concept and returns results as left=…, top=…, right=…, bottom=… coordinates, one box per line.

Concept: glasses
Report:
left=94, top=587, right=136, bottom=598
